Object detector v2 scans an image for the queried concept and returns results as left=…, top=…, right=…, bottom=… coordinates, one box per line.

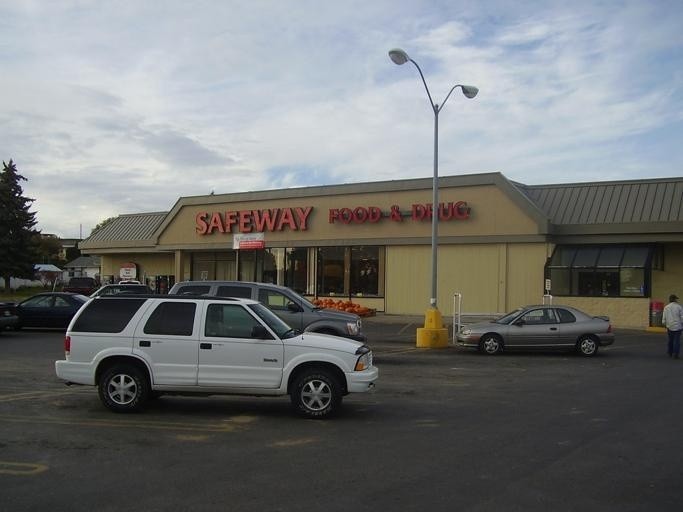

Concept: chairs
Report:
left=212, top=311, right=223, bottom=335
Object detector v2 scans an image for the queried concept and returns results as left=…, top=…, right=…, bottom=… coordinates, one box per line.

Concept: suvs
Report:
left=55, top=292, right=377, bottom=420
left=169, top=280, right=368, bottom=347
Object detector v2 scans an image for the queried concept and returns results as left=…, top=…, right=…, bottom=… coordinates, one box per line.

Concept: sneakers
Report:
left=667, top=353, right=680, bottom=360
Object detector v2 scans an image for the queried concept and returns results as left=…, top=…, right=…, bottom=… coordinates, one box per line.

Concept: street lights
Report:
left=389, top=48, right=479, bottom=306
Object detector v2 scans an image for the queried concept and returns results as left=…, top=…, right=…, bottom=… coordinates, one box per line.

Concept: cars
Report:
left=0, top=277, right=150, bottom=333
left=456, top=304, right=615, bottom=357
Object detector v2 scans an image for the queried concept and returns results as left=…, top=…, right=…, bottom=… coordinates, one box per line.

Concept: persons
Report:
left=661, top=294, right=682, bottom=359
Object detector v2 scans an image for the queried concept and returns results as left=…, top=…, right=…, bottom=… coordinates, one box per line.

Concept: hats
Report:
left=669, top=294, right=679, bottom=299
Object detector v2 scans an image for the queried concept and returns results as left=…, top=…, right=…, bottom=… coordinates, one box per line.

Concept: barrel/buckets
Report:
left=650, top=311, right=663, bottom=327
left=651, top=301, right=663, bottom=311
